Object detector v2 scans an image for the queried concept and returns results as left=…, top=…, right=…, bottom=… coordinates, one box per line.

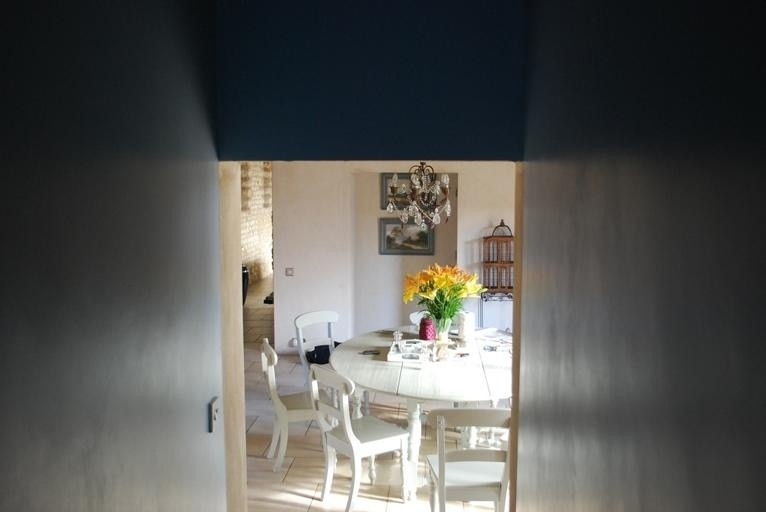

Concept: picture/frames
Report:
left=377, top=217, right=435, bottom=255
left=380, top=173, right=436, bottom=209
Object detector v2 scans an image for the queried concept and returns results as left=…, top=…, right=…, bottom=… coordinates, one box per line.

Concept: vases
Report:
left=435, top=319, right=453, bottom=339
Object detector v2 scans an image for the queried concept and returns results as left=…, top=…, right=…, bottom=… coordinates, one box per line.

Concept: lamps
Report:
left=387, top=160, right=452, bottom=230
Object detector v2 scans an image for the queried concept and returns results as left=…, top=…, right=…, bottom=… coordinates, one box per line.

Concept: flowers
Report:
left=403, top=264, right=482, bottom=319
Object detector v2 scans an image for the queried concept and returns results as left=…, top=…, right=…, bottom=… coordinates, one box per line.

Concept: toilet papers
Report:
left=457, top=310, right=474, bottom=340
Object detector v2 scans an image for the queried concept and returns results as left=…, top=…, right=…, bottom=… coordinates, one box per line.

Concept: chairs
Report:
left=427, top=409, right=510, bottom=512
left=294, top=308, right=370, bottom=416
left=410, top=311, right=467, bottom=332
left=309, top=363, right=411, bottom=512
left=259, top=338, right=331, bottom=471
